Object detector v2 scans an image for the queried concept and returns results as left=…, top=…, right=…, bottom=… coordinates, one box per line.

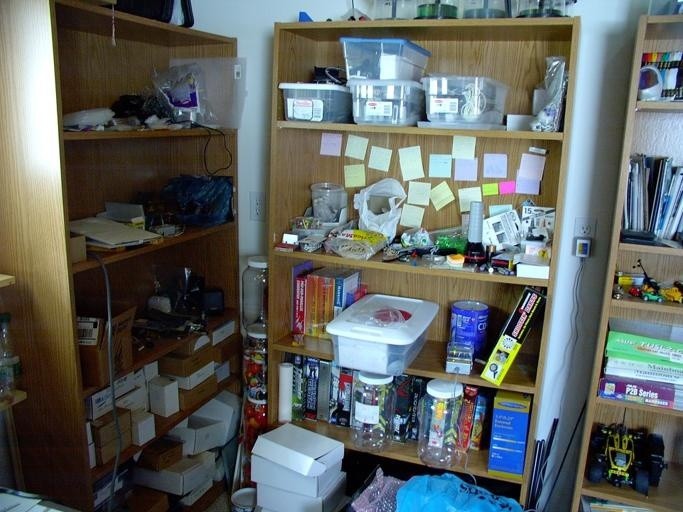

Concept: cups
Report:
left=369, top=0, right=574, bottom=19
left=309, top=181, right=343, bottom=222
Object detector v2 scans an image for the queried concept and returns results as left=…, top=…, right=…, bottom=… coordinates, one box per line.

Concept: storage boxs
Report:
left=325, top=292, right=439, bottom=374
left=78, top=297, right=236, bottom=511
left=249, top=422, right=351, bottom=512
left=271, top=30, right=513, bottom=127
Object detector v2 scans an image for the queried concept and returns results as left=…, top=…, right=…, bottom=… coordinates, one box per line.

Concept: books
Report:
left=620, top=149, right=682, bottom=247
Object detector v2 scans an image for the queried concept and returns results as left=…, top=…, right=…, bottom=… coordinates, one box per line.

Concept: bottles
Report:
left=243, top=390, right=268, bottom=458
left=241, top=255, right=269, bottom=335
left=351, top=370, right=395, bottom=453
left=243, top=323, right=268, bottom=394
left=418, top=380, right=465, bottom=467
left=1, top=314, right=17, bottom=403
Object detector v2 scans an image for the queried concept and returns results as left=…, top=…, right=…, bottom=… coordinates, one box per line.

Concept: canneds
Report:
left=448, top=299, right=490, bottom=359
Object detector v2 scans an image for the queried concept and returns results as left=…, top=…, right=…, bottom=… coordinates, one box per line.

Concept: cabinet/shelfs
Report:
left=0, top=273, right=30, bottom=492
left=565, top=15, right=683, bottom=512
left=0, top=1, right=245, bottom=512
left=269, top=16, right=579, bottom=512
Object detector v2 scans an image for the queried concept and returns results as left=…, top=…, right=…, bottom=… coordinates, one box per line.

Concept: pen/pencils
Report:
left=479, top=260, right=515, bottom=276
